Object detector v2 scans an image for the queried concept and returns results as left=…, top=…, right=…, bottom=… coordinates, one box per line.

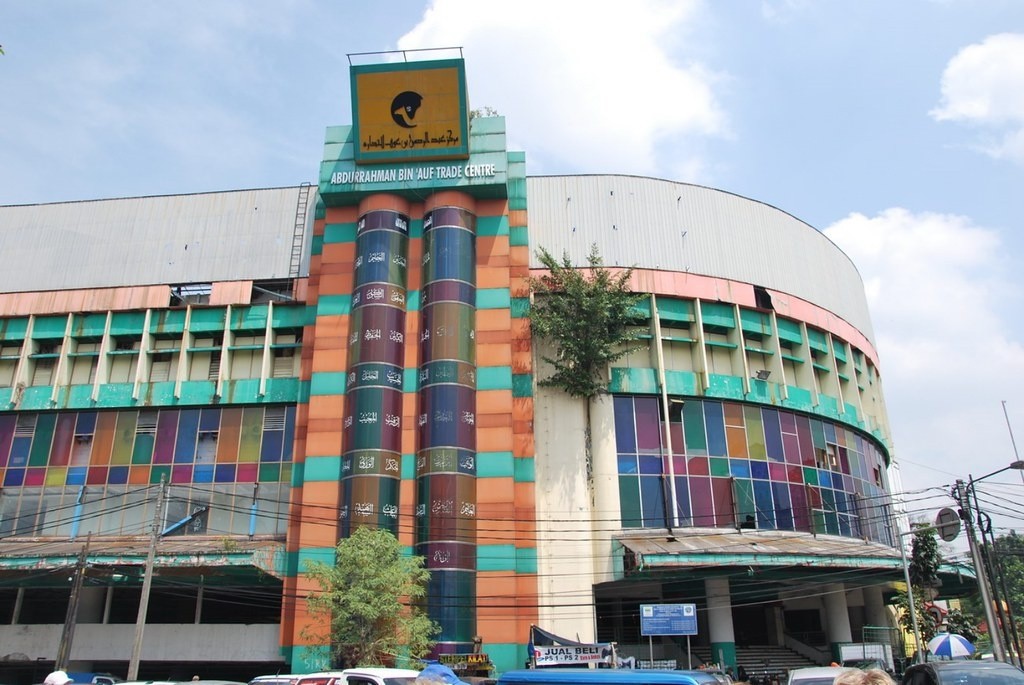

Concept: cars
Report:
left=496, top=661, right=1024, bottom=685
left=90, top=667, right=421, bottom=685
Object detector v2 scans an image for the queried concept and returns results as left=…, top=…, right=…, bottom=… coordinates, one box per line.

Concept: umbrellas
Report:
left=928, top=632, right=975, bottom=657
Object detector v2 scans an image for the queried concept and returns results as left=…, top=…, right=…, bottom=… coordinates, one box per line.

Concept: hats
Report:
left=44, top=670, right=73, bottom=685
left=417, top=663, right=471, bottom=685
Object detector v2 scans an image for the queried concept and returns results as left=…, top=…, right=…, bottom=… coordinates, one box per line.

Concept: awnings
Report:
left=613, top=534, right=980, bottom=599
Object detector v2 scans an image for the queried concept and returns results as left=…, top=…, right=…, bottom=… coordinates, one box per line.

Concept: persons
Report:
left=43, top=671, right=74, bottom=685
left=728, top=667, right=737, bottom=683
left=738, top=666, right=749, bottom=685
left=416, top=664, right=470, bottom=685
left=834, top=668, right=894, bottom=685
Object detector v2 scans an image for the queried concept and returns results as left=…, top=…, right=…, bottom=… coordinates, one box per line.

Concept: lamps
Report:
left=756, top=370, right=771, bottom=380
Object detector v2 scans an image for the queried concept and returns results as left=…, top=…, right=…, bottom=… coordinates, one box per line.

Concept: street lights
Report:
left=966, top=460, right=1024, bottom=664
